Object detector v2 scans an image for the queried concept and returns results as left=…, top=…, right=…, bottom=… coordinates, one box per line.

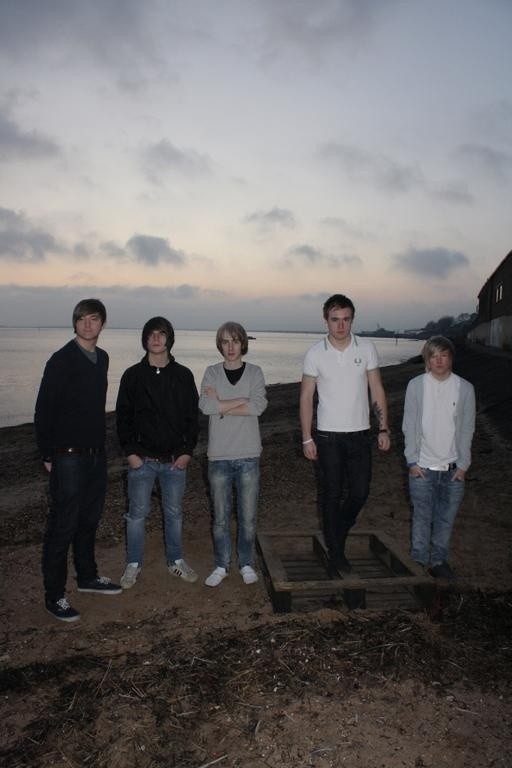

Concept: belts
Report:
left=141, top=453, right=179, bottom=465
left=56, top=447, right=104, bottom=454
left=428, top=462, right=457, bottom=471
left=316, top=429, right=371, bottom=439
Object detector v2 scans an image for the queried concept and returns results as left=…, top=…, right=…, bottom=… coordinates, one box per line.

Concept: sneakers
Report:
left=417, top=561, right=451, bottom=579
left=165, top=559, right=197, bottom=582
left=240, top=565, right=259, bottom=584
left=120, top=561, right=141, bottom=588
left=206, top=566, right=229, bottom=586
left=330, top=541, right=351, bottom=572
left=77, top=576, right=122, bottom=594
left=45, top=597, right=80, bottom=621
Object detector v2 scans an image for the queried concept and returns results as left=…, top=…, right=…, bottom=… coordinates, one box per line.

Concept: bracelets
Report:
left=407, top=462, right=418, bottom=468
left=377, top=428, right=392, bottom=436
left=302, top=437, right=314, bottom=445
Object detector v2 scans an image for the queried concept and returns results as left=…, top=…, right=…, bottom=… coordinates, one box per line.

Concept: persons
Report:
left=116, top=315, right=199, bottom=589
left=299, top=294, right=392, bottom=573
left=199, top=321, right=269, bottom=587
left=400, top=333, right=478, bottom=581
left=33, top=299, right=123, bottom=625
